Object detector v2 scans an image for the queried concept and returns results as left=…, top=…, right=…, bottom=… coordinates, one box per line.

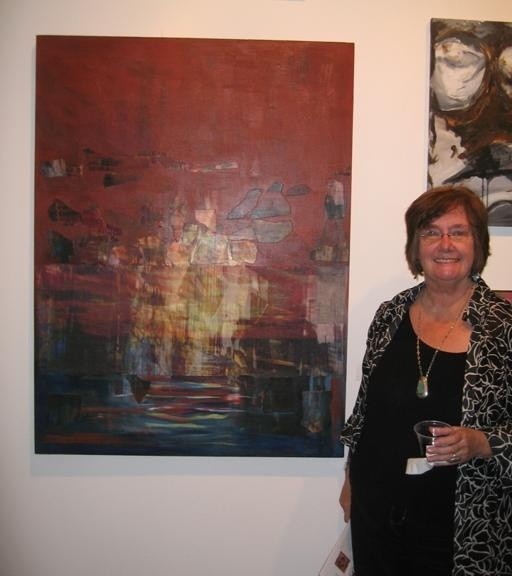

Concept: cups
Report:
left=412, top=420, right=452, bottom=458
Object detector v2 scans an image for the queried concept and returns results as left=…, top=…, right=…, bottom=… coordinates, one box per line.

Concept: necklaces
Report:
left=414, top=282, right=475, bottom=400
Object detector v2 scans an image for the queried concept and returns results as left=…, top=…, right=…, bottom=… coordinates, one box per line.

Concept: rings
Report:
left=447, top=453, right=457, bottom=464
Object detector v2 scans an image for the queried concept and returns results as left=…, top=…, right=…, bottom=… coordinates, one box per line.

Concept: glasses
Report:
left=417, top=228, right=469, bottom=244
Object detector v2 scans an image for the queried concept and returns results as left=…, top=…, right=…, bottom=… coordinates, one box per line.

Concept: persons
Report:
left=338, top=185, right=511, bottom=575
